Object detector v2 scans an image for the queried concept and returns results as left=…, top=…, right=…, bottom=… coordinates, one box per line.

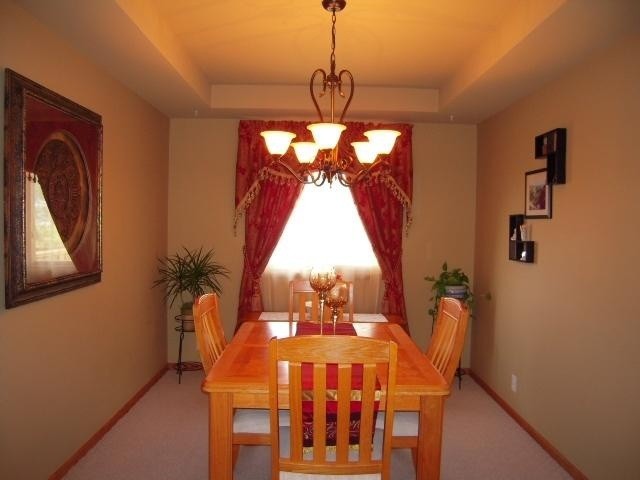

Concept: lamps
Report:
left=261, top=1, right=402, bottom=191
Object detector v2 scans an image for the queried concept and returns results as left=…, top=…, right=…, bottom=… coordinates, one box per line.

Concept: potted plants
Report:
left=425, top=261, right=491, bottom=320
left=152, top=244, right=232, bottom=331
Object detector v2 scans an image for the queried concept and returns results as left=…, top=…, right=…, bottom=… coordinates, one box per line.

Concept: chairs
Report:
left=290, top=279, right=354, bottom=315
left=268, top=334, right=398, bottom=479
left=193, top=292, right=231, bottom=377
left=426, top=297, right=469, bottom=386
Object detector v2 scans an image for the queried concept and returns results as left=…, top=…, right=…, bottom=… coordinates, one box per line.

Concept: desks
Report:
left=201, top=320, right=452, bottom=480
left=173, top=315, right=204, bottom=383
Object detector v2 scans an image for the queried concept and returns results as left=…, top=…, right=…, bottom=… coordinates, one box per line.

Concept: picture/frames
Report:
left=3, top=67, right=104, bottom=310
left=524, top=168, right=553, bottom=219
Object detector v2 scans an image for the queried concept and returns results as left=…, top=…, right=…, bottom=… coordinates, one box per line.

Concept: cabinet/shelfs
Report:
left=535, top=128, right=567, bottom=184
left=509, top=214, right=535, bottom=263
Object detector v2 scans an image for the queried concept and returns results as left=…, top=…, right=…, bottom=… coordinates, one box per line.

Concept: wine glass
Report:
left=323, top=281, right=348, bottom=340
left=307, top=263, right=338, bottom=338
